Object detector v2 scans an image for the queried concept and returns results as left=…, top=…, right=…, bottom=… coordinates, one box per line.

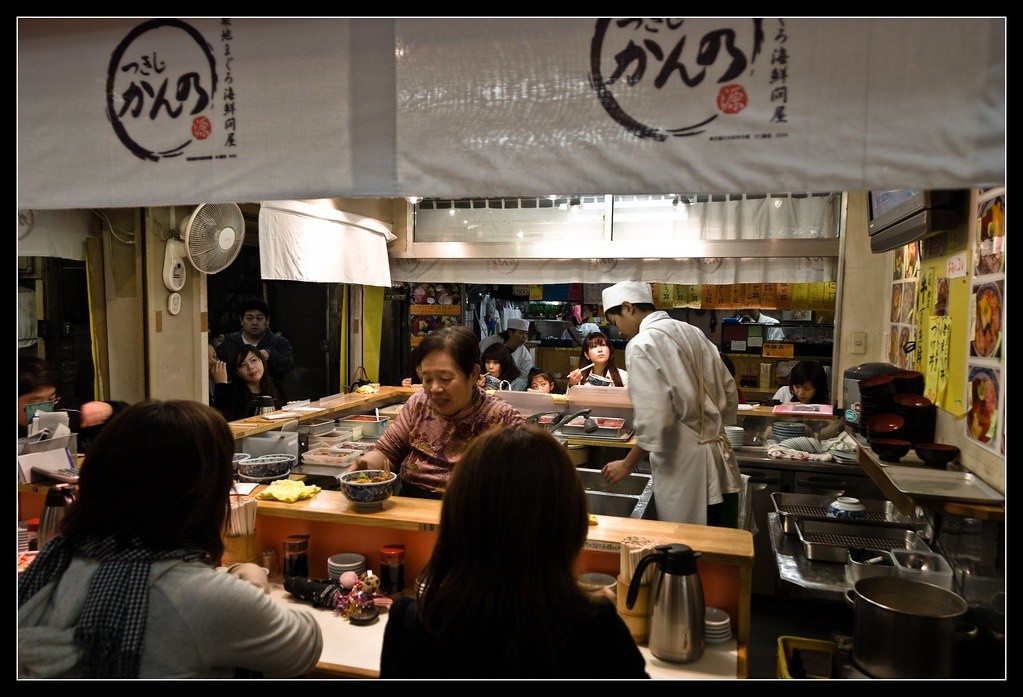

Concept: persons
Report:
left=722, top=355, right=746, bottom=404
left=381, top=427, right=651, bottom=679
left=563, top=315, right=601, bottom=345
left=773, top=360, right=829, bottom=405
left=19, top=355, right=131, bottom=454
left=346, top=328, right=527, bottom=500
left=738, top=309, right=785, bottom=341
left=401, top=364, right=423, bottom=387
left=478, top=342, right=527, bottom=391
left=565, top=332, right=629, bottom=394
left=602, top=281, right=744, bottom=530
left=209, top=300, right=293, bottom=421
left=480, top=318, right=533, bottom=390
left=529, top=368, right=555, bottom=394
left=19, top=398, right=322, bottom=679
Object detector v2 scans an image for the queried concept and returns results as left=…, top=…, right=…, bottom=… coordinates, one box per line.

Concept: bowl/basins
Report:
left=485, top=375, right=504, bottom=390
left=858, top=371, right=960, bottom=470
left=901, top=553, right=935, bottom=571
left=340, top=469, right=397, bottom=509
left=825, top=496, right=867, bottom=520
left=230, top=452, right=297, bottom=485
left=588, top=373, right=612, bottom=386
left=967, top=194, right=1009, bottom=444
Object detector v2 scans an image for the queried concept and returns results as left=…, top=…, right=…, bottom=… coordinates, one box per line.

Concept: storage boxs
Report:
left=240, top=418, right=299, bottom=467
left=18, top=431, right=80, bottom=480
left=776, top=636, right=834, bottom=680
left=298, top=448, right=361, bottom=469
left=377, top=403, right=405, bottom=418
left=307, top=430, right=353, bottom=449
left=567, top=380, right=635, bottom=425
left=331, top=442, right=378, bottom=455
left=338, top=412, right=391, bottom=439
left=335, top=423, right=364, bottom=441
left=296, top=418, right=336, bottom=434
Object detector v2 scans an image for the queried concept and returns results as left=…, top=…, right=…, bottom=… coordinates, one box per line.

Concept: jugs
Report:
left=246, top=395, right=277, bottom=417
left=37, top=483, right=78, bottom=550
left=626, top=543, right=705, bottom=663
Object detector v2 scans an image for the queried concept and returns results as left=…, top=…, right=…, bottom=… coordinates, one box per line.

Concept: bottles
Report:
left=378, top=544, right=407, bottom=595
left=412, top=558, right=432, bottom=598
left=260, top=548, right=279, bottom=580
left=282, top=534, right=311, bottom=580
left=27, top=518, right=40, bottom=551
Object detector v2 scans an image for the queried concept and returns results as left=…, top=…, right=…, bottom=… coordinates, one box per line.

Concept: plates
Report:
left=552, top=372, right=562, bottom=378
left=578, top=572, right=618, bottom=600
left=773, top=422, right=822, bottom=453
left=327, top=553, right=366, bottom=582
left=18, top=528, right=29, bottom=552
left=723, top=426, right=744, bottom=449
left=703, top=606, right=732, bottom=643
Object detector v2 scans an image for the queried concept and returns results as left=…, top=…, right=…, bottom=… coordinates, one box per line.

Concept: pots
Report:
left=844, top=577, right=969, bottom=679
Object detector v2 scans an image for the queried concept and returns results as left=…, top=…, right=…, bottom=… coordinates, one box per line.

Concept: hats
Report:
left=601, top=281, right=653, bottom=315
left=508, top=318, right=530, bottom=331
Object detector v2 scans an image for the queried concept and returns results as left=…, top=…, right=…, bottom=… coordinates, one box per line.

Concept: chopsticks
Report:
left=566, top=357, right=595, bottom=377
left=760, top=362, right=771, bottom=374
left=224, top=496, right=258, bottom=537
left=619, top=534, right=659, bottom=584
left=482, top=372, right=490, bottom=377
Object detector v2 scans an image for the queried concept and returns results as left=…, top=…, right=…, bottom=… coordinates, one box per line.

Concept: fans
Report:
left=160, top=201, right=250, bottom=285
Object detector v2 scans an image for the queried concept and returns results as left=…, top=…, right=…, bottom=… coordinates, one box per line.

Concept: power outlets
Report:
left=167, top=292, right=183, bottom=315
